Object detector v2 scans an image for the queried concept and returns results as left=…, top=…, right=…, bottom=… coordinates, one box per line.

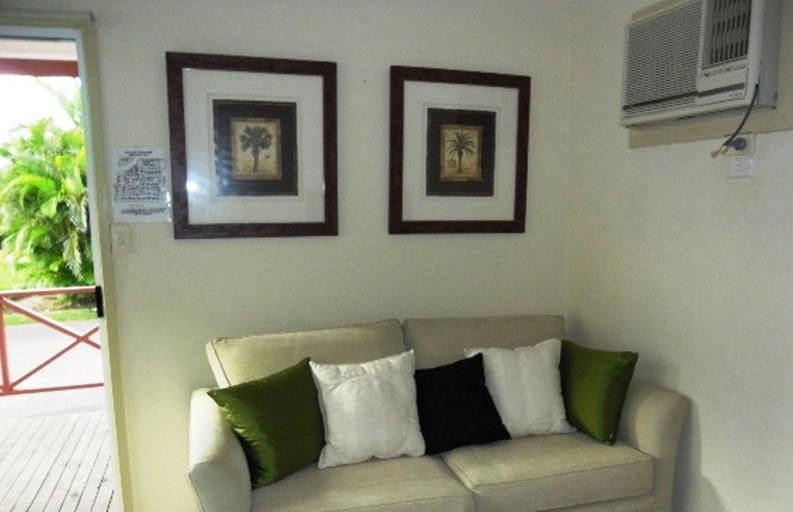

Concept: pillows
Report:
left=559, top=338, right=640, bottom=444
left=463, top=336, right=580, bottom=438
left=413, top=351, right=511, bottom=454
left=203, top=354, right=326, bottom=491
left=305, top=346, right=424, bottom=469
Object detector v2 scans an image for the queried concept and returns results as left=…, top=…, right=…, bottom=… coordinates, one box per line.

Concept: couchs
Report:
left=185, top=311, right=691, bottom=512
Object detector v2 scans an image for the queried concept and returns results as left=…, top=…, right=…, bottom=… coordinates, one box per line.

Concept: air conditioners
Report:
left=616, top=0, right=780, bottom=129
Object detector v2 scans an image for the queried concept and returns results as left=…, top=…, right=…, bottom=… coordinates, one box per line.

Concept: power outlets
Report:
left=723, top=130, right=752, bottom=157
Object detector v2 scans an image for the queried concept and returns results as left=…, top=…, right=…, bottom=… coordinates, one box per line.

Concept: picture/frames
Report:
left=164, top=50, right=339, bottom=240
left=386, top=63, right=532, bottom=238
